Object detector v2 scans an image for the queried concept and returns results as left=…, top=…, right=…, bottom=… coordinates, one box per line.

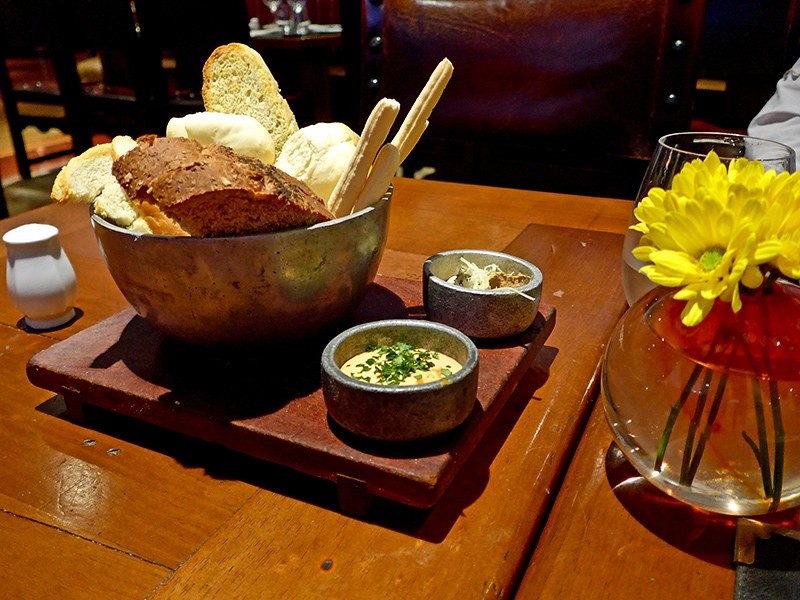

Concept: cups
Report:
left=620, top=131, right=797, bottom=309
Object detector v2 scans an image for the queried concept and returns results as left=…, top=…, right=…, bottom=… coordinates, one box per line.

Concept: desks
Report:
left=248, top=24, right=345, bottom=127
left=0, top=176, right=800, bottom=599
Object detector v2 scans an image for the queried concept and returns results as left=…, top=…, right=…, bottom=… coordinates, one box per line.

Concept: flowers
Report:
left=630, top=149, right=799, bottom=511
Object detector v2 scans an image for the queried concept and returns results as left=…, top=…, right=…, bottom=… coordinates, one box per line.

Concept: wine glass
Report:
left=263, top=0, right=308, bottom=36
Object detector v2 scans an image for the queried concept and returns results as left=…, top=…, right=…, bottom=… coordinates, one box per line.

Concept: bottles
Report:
left=3, top=222, right=79, bottom=329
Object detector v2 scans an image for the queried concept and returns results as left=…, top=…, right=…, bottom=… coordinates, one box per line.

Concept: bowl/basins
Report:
left=321, top=319, right=479, bottom=439
left=88, top=183, right=394, bottom=345
left=422, top=249, right=545, bottom=337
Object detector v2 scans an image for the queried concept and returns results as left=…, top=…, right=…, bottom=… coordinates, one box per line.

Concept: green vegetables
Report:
left=343, top=340, right=452, bottom=387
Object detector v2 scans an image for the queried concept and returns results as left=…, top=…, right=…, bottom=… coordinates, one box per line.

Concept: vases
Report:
left=603, top=267, right=798, bottom=517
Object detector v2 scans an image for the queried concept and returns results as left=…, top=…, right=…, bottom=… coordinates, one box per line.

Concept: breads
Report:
left=50, top=43, right=453, bottom=237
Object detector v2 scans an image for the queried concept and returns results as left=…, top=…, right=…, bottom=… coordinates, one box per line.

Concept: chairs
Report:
left=0, top=0, right=368, bottom=181
left=382, top=0, right=671, bottom=200
